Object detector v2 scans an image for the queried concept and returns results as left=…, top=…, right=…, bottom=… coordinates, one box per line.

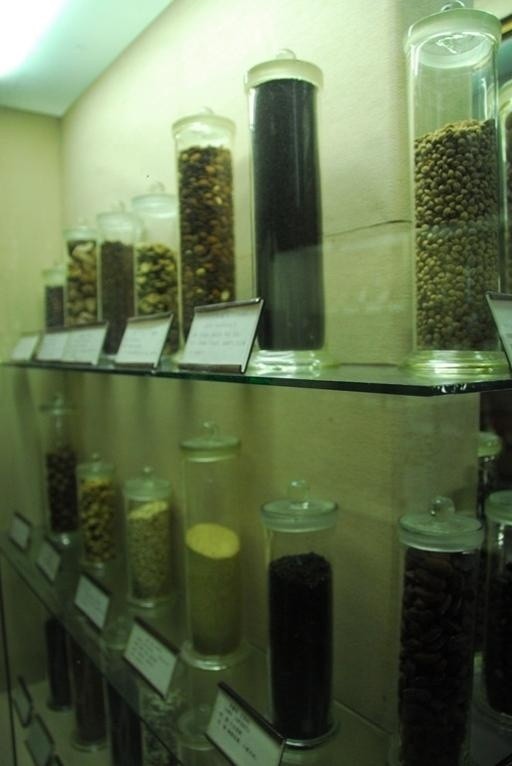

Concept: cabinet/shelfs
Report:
left=3, top=333, right=510, bottom=759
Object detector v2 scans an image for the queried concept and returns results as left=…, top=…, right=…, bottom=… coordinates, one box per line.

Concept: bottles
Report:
left=405, top=2, right=512, bottom=376
left=398, top=494, right=491, bottom=763
left=38, top=184, right=181, bottom=359
left=243, top=50, right=334, bottom=378
left=477, top=427, right=512, bottom=556
left=35, top=614, right=218, bottom=766
left=262, top=483, right=345, bottom=753
left=41, top=394, right=180, bottom=609
left=172, top=108, right=239, bottom=355
left=180, top=434, right=251, bottom=671
